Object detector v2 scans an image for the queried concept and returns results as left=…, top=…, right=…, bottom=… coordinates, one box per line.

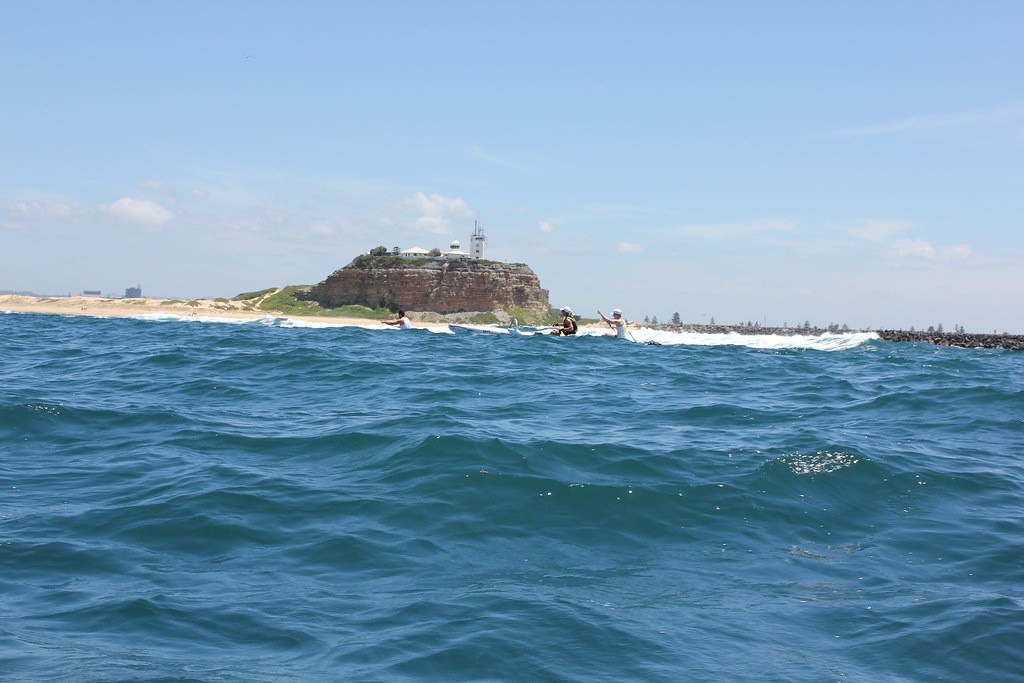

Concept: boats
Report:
left=448, top=318, right=580, bottom=336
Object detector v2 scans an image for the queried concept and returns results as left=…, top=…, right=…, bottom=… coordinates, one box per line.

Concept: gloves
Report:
left=559, top=328, right=564, bottom=334
left=552, top=323, right=559, bottom=327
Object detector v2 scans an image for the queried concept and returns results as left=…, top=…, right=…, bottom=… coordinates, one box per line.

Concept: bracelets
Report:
left=601, top=313, right=602, bottom=316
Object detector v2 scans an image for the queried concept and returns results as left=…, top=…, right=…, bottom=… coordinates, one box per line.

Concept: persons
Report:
left=381, top=310, right=410, bottom=329
left=598, top=309, right=627, bottom=338
left=552, top=306, right=576, bottom=335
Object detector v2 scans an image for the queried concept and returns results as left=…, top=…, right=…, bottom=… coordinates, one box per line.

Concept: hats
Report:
left=560, top=307, right=570, bottom=314
left=610, top=309, right=622, bottom=315
